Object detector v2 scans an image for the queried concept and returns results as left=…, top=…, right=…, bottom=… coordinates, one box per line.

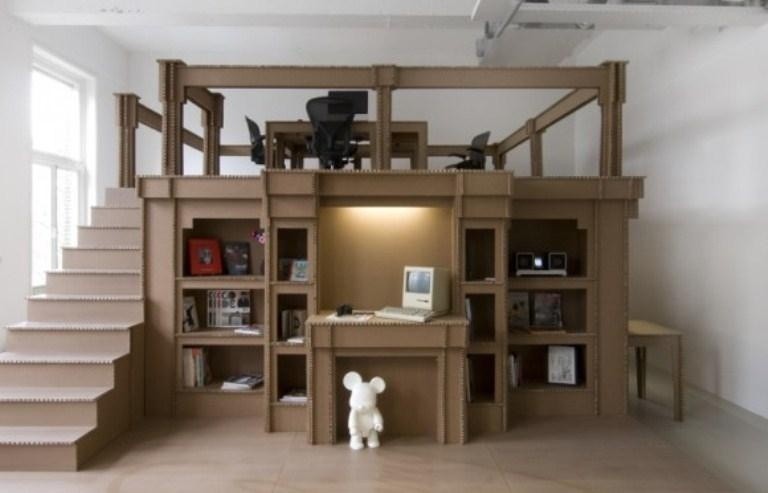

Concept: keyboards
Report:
left=374, top=306, right=433, bottom=323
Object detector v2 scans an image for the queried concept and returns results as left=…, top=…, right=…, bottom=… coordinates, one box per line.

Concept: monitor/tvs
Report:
left=401, top=265, right=451, bottom=316
left=328, top=90, right=368, bottom=114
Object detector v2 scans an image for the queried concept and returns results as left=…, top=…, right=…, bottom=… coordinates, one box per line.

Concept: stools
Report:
left=629, top=320, right=686, bottom=422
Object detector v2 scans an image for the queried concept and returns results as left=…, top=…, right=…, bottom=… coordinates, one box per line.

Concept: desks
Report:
left=266, top=119, right=428, bottom=171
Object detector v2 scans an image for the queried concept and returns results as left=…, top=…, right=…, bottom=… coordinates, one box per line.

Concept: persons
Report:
left=199, top=248, right=212, bottom=264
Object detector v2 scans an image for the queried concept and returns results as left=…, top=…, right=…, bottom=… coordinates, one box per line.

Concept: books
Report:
left=183, top=297, right=200, bottom=332
left=279, top=390, right=309, bottom=403
left=206, top=290, right=251, bottom=329
left=465, top=268, right=577, bottom=403
left=182, top=348, right=211, bottom=386
left=222, top=374, right=264, bottom=391
left=281, top=308, right=306, bottom=338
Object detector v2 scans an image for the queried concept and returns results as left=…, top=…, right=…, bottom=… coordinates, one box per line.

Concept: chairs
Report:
left=303, top=96, right=361, bottom=169
left=244, top=114, right=267, bottom=165
left=445, top=130, right=492, bottom=170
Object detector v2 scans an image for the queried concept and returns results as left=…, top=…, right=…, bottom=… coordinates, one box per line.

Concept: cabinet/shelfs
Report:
left=142, top=169, right=646, bottom=445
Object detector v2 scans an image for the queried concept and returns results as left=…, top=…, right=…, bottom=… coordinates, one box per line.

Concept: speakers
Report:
left=544, top=250, right=567, bottom=270
left=515, top=251, right=534, bottom=270
